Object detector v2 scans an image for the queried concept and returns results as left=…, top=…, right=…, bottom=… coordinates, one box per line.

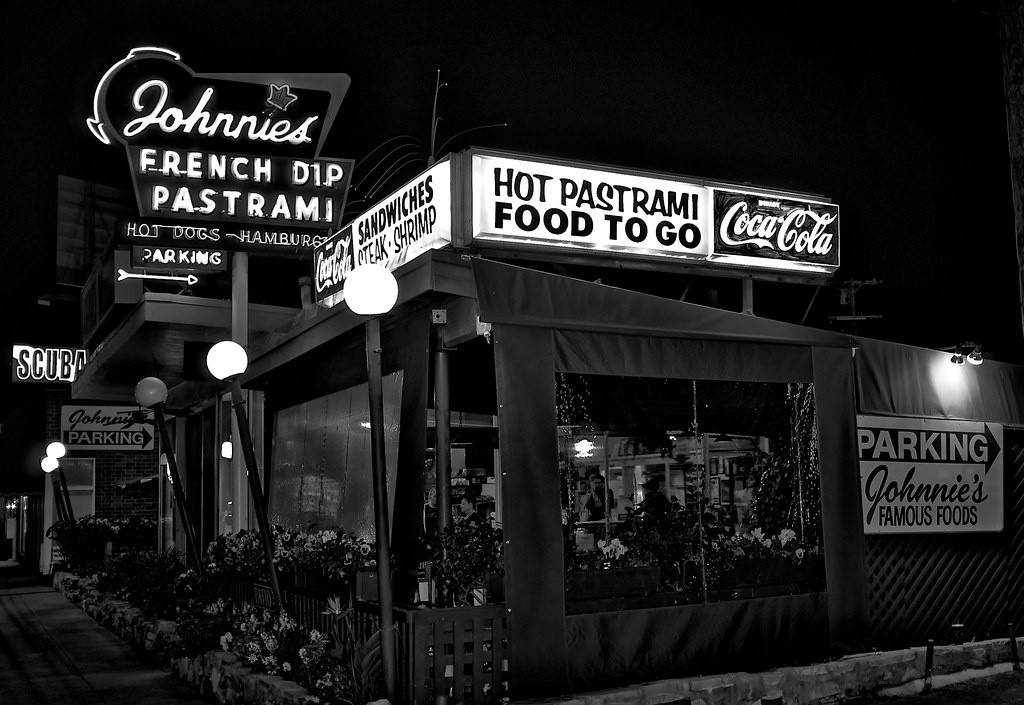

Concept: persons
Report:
left=584, top=474, right=617, bottom=521
left=423, top=487, right=439, bottom=549
left=458, top=494, right=484, bottom=524
left=634, top=479, right=669, bottom=517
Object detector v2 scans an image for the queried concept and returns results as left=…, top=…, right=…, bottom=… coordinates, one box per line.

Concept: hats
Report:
left=642, top=478, right=659, bottom=491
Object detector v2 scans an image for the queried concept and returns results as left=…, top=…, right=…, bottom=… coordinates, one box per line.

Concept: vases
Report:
left=294, top=563, right=396, bottom=606
left=565, top=563, right=660, bottom=603
left=767, top=557, right=821, bottom=584
left=486, top=571, right=510, bottom=604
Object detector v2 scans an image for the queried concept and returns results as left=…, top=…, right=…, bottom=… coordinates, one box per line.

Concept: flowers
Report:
left=202, top=521, right=377, bottom=574
left=170, top=570, right=336, bottom=695
left=713, top=516, right=806, bottom=547
left=581, top=520, right=652, bottom=565
left=436, top=509, right=505, bottom=570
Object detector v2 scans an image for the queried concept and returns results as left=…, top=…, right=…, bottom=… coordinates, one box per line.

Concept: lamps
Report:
left=221, top=432, right=235, bottom=464
left=714, top=431, right=734, bottom=444
left=939, top=341, right=985, bottom=366
left=950, top=623, right=964, bottom=645
